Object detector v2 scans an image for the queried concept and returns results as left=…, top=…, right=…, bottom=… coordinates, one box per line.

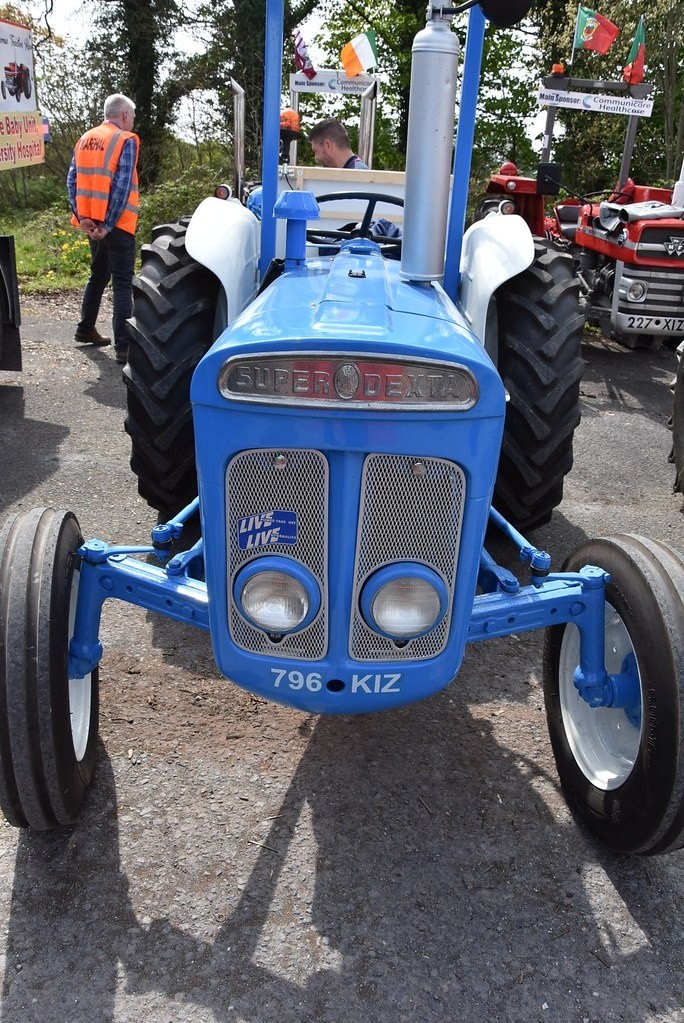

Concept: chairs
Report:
left=552, top=199, right=577, bottom=242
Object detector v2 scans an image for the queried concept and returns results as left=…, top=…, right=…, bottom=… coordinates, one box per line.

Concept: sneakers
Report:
left=116, top=350, right=128, bottom=363
left=76, top=327, right=110, bottom=344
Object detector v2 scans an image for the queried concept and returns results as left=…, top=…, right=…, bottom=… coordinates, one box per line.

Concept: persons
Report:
left=67, top=94, right=140, bottom=362
left=309, top=118, right=370, bottom=170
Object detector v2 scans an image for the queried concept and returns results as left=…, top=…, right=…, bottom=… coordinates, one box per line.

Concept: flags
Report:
left=625, top=19, right=645, bottom=85
left=341, top=30, right=378, bottom=79
left=293, top=30, right=317, bottom=81
left=574, top=5, right=619, bottom=54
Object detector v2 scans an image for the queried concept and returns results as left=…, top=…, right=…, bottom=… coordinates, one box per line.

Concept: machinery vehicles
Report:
left=1, top=0, right=684, bottom=857
left=474, top=2, right=684, bottom=352
